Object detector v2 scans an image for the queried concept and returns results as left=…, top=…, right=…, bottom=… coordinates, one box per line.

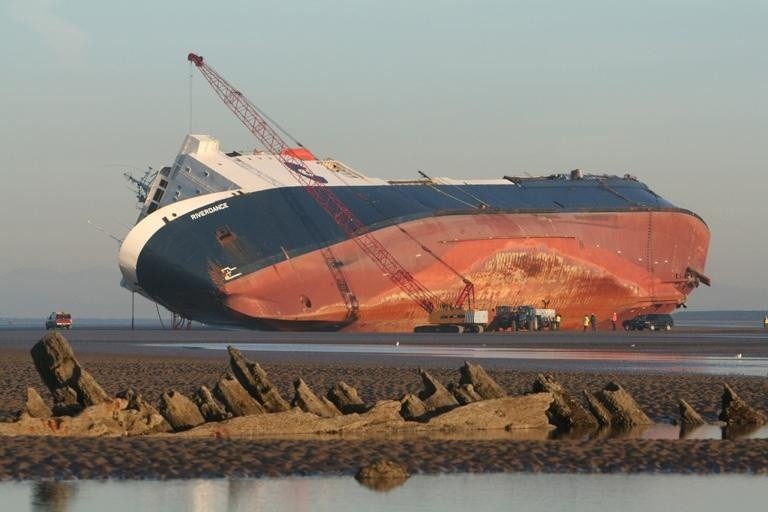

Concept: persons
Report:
left=610, top=312, right=617, bottom=330
left=555, top=313, right=562, bottom=330
left=590, top=312, right=597, bottom=331
left=583, top=314, right=590, bottom=332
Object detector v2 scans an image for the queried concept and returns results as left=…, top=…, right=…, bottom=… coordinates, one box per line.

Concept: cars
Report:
left=622, top=314, right=674, bottom=331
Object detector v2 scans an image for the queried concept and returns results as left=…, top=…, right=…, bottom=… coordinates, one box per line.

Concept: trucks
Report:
left=495, top=306, right=557, bottom=331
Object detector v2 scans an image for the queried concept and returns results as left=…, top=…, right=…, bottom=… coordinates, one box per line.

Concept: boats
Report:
left=86, top=134, right=711, bottom=333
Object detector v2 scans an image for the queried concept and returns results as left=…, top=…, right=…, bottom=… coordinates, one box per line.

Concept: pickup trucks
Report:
left=46, top=312, right=72, bottom=330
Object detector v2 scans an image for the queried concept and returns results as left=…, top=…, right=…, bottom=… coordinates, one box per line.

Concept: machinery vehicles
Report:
left=187, top=52, right=489, bottom=333
